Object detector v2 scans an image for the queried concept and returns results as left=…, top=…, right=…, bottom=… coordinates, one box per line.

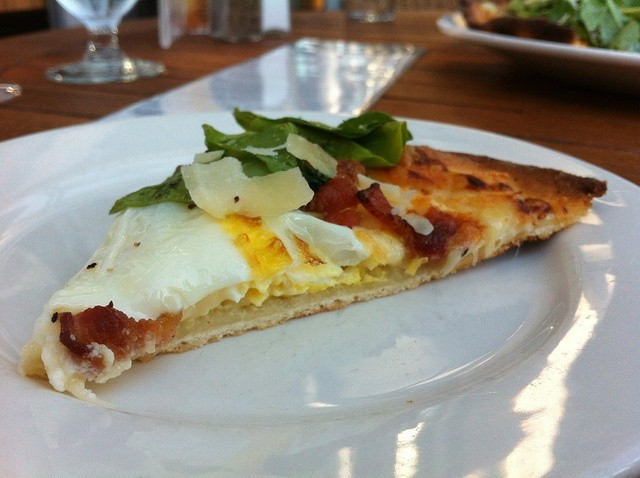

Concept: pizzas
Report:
left=15, top=108, right=607, bottom=404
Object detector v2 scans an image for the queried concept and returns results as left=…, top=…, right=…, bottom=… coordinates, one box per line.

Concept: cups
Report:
left=348, top=2, right=396, bottom=23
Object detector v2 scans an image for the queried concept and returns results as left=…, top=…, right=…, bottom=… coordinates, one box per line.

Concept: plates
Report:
left=3, top=109, right=639, bottom=478
left=433, top=6, right=640, bottom=87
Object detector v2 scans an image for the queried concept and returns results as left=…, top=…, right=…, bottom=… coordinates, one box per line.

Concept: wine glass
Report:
left=45, top=0, right=167, bottom=83
